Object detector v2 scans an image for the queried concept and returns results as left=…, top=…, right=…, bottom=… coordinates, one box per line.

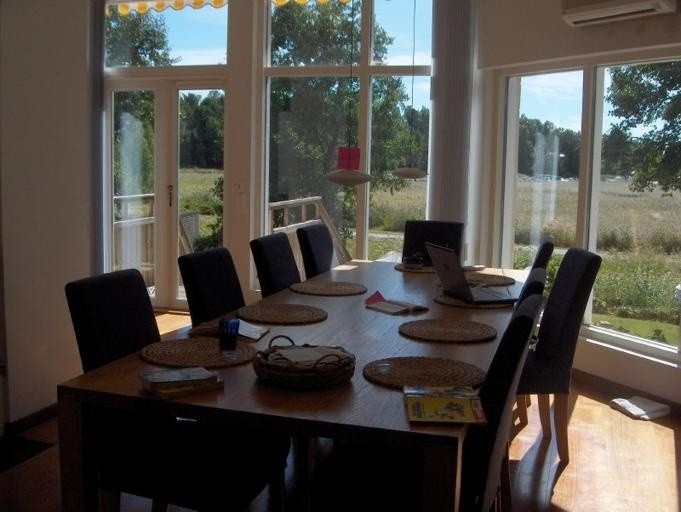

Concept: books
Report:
left=138, top=366, right=224, bottom=398
left=402, top=386, right=488, bottom=424
left=365, top=299, right=429, bottom=315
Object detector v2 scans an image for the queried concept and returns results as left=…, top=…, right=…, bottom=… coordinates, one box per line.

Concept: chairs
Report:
left=177, top=222, right=342, bottom=326
left=66, top=269, right=292, bottom=510
left=402, top=219, right=465, bottom=267
left=459, top=242, right=602, bottom=512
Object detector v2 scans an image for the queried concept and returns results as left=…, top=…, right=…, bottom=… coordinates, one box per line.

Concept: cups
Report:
left=218, top=318, right=240, bottom=351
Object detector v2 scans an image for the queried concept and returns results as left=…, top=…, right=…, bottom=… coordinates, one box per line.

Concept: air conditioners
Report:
left=561, top=0, right=678, bottom=27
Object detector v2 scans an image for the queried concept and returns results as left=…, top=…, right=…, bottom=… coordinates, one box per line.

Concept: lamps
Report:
left=394, top=167, right=427, bottom=179
left=324, top=167, right=374, bottom=186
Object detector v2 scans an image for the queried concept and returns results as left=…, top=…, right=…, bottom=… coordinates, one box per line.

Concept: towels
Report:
left=611, top=396, right=672, bottom=421
